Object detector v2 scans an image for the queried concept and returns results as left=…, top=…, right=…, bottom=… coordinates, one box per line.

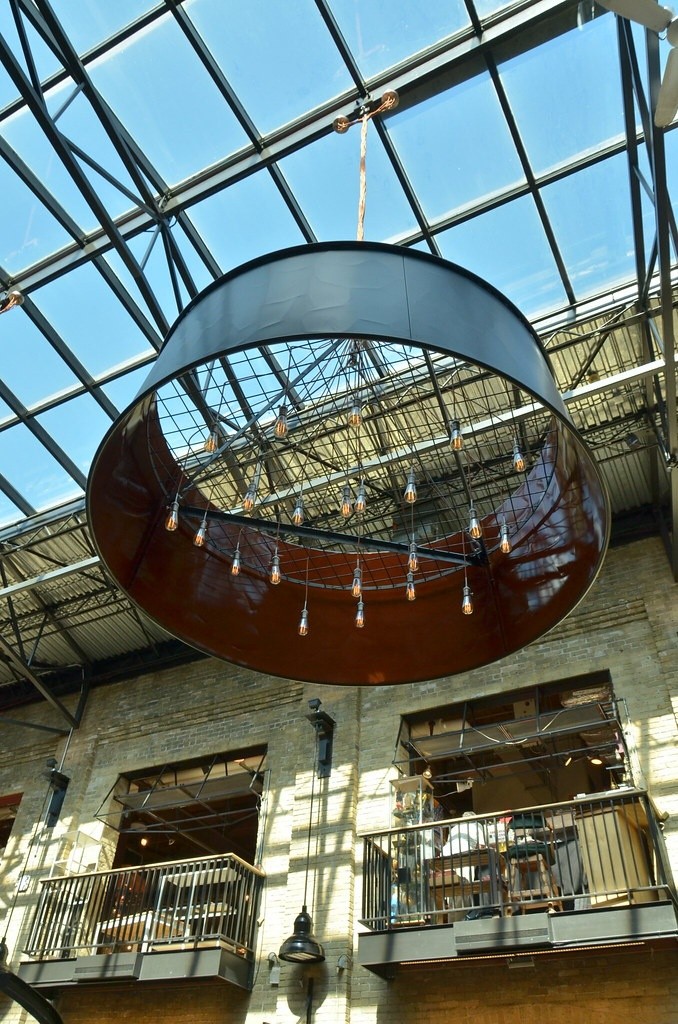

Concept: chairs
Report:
left=504, top=813, right=565, bottom=917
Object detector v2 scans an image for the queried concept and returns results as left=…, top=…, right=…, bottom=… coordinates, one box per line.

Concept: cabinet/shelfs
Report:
left=425, top=848, right=506, bottom=924
left=575, top=805, right=659, bottom=907
left=385, top=775, right=436, bottom=926
left=145, top=866, right=246, bottom=953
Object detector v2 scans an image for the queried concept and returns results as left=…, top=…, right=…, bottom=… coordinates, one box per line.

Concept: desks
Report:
left=91, top=910, right=191, bottom=955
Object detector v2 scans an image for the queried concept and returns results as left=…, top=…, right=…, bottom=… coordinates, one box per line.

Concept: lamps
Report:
left=587, top=749, right=602, bottom=765
left=279, top=698, right=336, bottom=965
left=85, top=88, right=611, bottom=688
left=423, top=758, right=433, bottom=779
left=166, top=834, right=175, bottom=845
left=557, top=755, right=572, bottom=767
left=0, top=759, right=70, bottom=974
